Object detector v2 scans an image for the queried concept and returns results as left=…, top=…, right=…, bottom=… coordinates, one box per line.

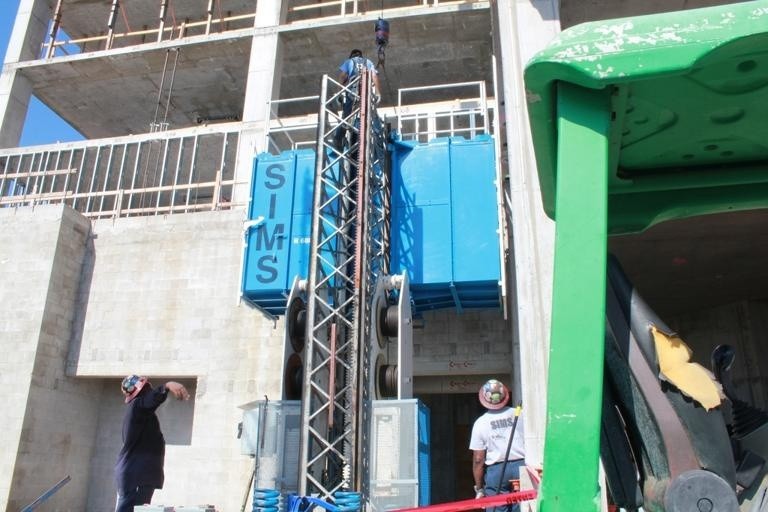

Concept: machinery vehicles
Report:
left=523, top=0, right=768, bottom=512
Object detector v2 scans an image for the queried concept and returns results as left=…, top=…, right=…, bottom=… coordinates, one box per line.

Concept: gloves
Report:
left=474, top=485, right=485, bottom=510
left=374, top=94, right=381, bottom=104
left=336, top=95, right=342, bottom=107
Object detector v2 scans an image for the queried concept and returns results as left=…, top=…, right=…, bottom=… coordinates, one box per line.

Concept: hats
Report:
left=350, top=49, right=362, bottom=58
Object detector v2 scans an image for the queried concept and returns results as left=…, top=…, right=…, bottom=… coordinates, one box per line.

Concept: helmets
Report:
left=121, top=375, right=149, bottom=404
left=479, top=379, right=510, bottom=410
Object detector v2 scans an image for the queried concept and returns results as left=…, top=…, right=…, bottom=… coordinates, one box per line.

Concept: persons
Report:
left=469, top=379, right=524, bottom=512
left=328, top=49, right=382, bottom=157
left=114, top=374, right=191, bottom=511
